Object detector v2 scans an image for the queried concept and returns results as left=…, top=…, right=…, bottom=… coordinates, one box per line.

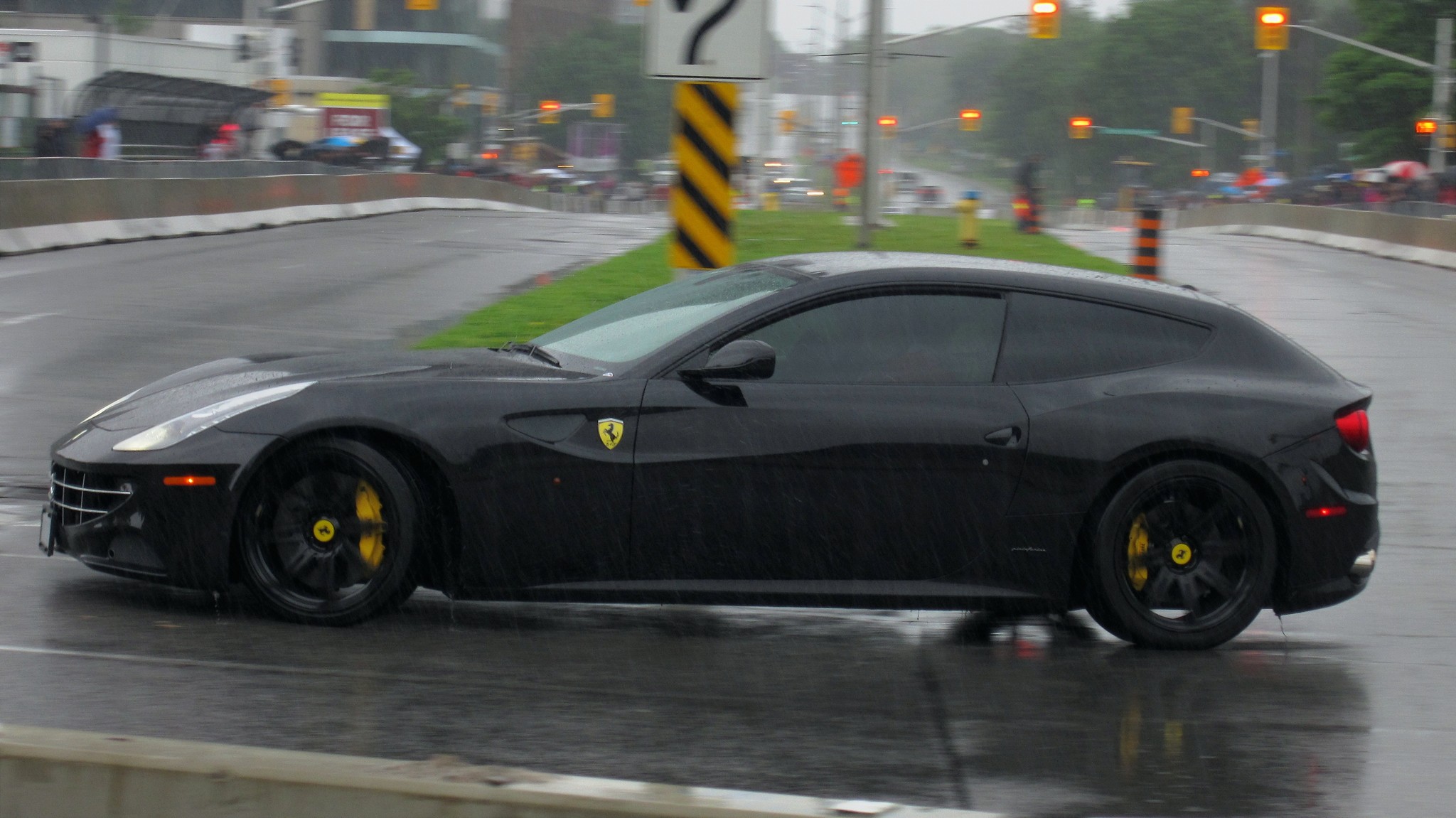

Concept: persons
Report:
left=835, top=153, right=865, bottom=212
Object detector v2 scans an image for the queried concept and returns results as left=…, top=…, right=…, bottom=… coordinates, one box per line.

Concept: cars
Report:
left=281, top=135, right=677, bottom=203
left=1172, top=159, right=1456, bottom=221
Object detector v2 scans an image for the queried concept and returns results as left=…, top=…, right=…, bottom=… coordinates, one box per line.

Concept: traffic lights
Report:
left=958, top=109, right=982, bottom=131
left=1029, top=0, right=1062, bottom=39
left=877, top=115, right=898, bottom=139
left=1413, top=119, right=1437, bottom=136
left=1253, top=6, right=1290, bottom=51
left=1068, top=118, right=1093, bottom=139
left=536, top=100, right=560, bottom=124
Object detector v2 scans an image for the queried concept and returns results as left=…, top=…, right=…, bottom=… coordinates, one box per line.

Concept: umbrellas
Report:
left=1221, top=161, right=1438, bottom=196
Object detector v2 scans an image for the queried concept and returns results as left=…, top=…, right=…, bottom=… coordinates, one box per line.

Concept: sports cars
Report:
left=35, top=250, right=1382, bottom=654
